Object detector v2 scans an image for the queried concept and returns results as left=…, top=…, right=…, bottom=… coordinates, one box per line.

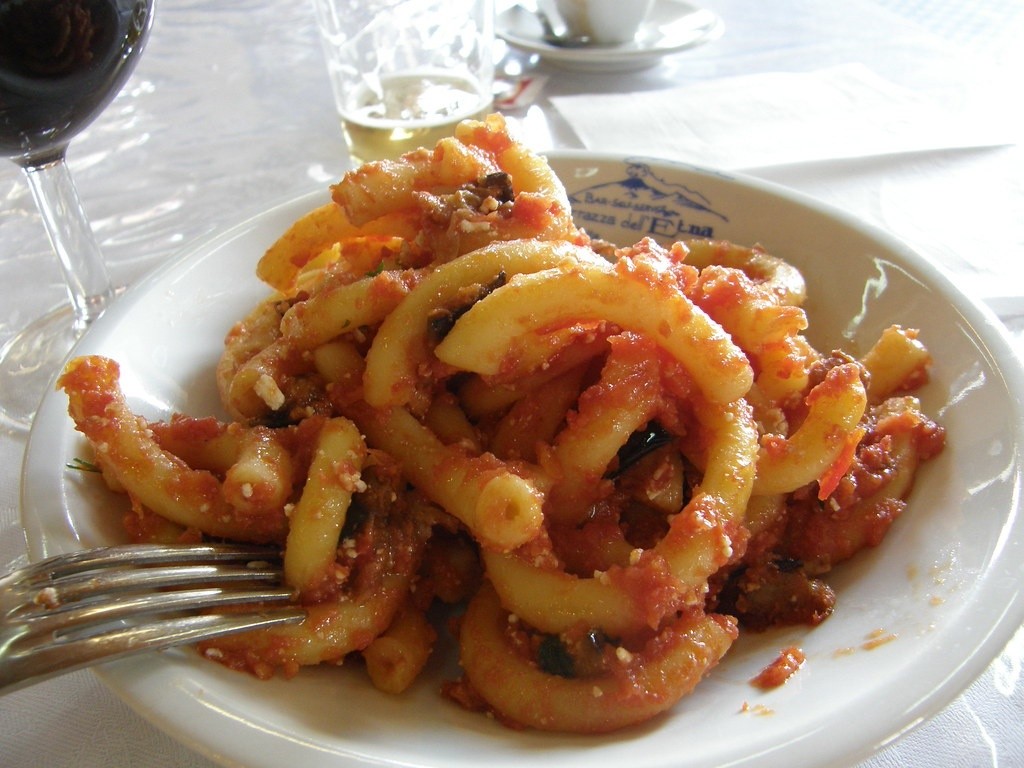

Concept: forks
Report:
left=1, top=540, right=308, bottom=686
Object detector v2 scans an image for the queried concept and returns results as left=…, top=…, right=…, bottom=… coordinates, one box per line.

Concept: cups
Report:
left=310, top=0, right=498, bottom=166
left=536, top=0, right=651, bottom=48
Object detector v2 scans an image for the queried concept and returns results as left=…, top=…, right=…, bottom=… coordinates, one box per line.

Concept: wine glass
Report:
left=0, top=0, right=158, bottom=438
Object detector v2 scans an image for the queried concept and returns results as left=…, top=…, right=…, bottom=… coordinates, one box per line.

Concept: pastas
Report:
left=52, top=109, right=931, bottom=738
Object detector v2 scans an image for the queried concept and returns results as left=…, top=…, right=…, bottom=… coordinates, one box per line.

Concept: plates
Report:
left=18, top=149, right=1023, bottom=768
left=495, top=0, right=726, bottom=74
